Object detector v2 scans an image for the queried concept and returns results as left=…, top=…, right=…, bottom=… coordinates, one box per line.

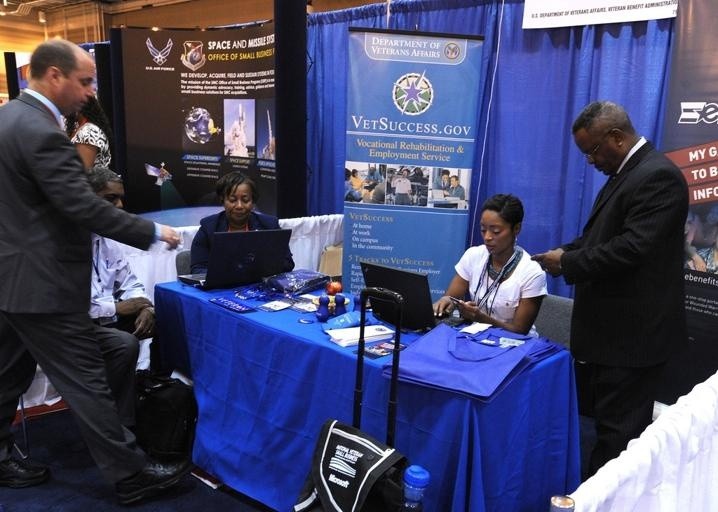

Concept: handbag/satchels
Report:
left=380, top=320, right=566, bottom=404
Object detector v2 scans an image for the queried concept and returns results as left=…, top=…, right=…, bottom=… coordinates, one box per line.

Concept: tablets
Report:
left=359, top=261, right=451, bottom=328
left=177, top=228, right=293, bottom=291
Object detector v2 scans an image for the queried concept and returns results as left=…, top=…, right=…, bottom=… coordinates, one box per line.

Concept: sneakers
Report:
left=0, top=458, right=52, bottom=489
left=112, top=462, right=186, bottom=505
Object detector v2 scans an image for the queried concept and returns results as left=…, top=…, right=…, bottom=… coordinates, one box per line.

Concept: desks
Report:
left=153, top=273, right=581, bottom=506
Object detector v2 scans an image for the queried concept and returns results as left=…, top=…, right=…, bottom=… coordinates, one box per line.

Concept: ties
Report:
left=452, top=187, right=456, bottom=195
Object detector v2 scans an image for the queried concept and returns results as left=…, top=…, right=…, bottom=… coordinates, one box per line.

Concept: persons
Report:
left=0, top=42, right=196, bottom=505
left=345, top=167, right=465, bottom=208
left=531, top=101, right=689, bottom=481
left=66, top=97, right=114, bottom=171
left=193, top=173, right=295, bottom=275
left=85, top=168, right=158, bottom=428
left=436, top=194, right=548, bottom=338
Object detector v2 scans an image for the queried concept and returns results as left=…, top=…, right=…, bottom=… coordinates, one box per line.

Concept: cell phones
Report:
left=448, top=296, right=460, bottom=303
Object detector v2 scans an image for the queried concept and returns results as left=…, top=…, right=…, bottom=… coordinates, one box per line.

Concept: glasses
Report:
left=585, top=129, right=615, bottom=159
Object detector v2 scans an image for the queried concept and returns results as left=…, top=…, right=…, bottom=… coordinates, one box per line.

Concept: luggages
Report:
left=127, top=371, right=199, bottom=469
left=291, top=287, right=423, bottom=512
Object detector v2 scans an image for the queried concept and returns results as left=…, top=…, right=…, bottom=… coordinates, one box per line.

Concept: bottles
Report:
left=401, top=466, right=429, bottom=511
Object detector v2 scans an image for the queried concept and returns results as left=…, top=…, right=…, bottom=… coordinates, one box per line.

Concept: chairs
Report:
left=536, top=295, right=575, bottom=352
